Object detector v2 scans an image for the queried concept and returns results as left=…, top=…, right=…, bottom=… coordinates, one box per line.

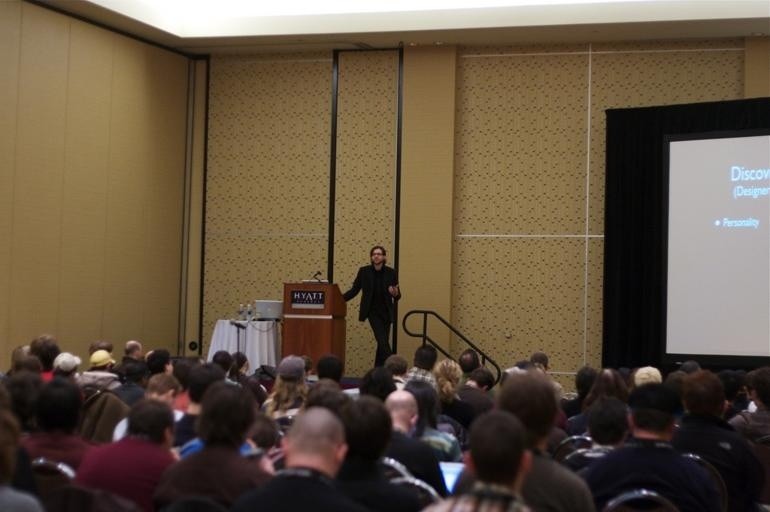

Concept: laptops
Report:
left=256, top=300, right=283, bottom=318
left=439, top=461, right=465, bottom=494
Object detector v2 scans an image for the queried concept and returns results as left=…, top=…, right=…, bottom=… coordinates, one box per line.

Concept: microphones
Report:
left=313, top=270, right=322, bottom=282
left=229, top=319, right=246, bottom=330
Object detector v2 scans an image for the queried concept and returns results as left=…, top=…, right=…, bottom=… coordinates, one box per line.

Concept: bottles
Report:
left=238, top=303, right=254, bottom=322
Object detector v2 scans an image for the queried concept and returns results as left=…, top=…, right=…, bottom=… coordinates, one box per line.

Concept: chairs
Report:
left=12, top=461, right=227, bottom=511
left=550, top=423, right=770, bottom=510
left=367, top=457, right=447, bottom=509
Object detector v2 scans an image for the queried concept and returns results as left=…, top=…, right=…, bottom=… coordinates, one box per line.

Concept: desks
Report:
left=203, top=316, right=282, bottom=377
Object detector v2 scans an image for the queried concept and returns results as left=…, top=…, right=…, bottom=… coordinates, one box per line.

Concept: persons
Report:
left=343, top=245, right=402, bottom=368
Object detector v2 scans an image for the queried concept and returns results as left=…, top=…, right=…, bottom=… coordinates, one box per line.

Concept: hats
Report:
left=54, top=351, right=82, bottom=373
left=278, top=353, right=309, bottom=377
left=89, top=348, right=116, bottom=369
